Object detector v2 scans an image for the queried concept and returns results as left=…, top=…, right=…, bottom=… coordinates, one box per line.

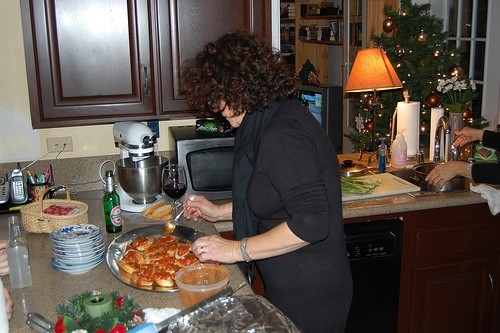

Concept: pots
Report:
left=408, top=162, right=465, bottom=193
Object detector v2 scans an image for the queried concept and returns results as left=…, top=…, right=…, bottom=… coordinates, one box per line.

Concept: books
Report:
left=351, top=0, right=363, bottom=46
left=280, top=4, right=342, bottom=56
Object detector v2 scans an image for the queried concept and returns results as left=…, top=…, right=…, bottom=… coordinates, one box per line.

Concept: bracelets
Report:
left=240, top=236, right=251, bottom=263
left=467, top=164, right=470, bottom=177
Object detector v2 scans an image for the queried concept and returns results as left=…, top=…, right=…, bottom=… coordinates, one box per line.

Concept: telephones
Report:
left=10, top=161, right=28, bottom=204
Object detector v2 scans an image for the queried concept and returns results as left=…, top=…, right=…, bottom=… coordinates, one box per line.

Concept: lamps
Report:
left=344, top=47, right=403, bottom=153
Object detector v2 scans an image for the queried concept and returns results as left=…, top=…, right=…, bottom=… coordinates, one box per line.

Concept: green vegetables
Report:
left=338, top=177, right=382, bottom=194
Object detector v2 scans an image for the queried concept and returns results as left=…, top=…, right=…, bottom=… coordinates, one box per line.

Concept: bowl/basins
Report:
left=114, top=157, right=170, bottom=203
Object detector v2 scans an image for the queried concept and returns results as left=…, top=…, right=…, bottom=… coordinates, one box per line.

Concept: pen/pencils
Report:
left=26, top=168, right=50, bottom=184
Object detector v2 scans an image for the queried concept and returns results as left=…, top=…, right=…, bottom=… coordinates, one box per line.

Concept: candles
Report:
left=396, top=91, right=421, bottom=159
left=429, top=107, right=445, bottom=159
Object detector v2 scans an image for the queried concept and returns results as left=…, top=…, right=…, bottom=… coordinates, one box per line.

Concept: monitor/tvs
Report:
left=297, top=83, right=344, bottom=154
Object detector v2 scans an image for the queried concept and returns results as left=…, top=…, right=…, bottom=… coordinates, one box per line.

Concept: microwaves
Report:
left=169, top=125, right=238, bottom=201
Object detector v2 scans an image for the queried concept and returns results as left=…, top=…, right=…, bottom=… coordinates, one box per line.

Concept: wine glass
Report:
left=161, top=163, right=187, bottom=225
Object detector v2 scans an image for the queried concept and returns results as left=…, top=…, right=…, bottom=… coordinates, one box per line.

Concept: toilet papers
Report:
left=396, top=100, right=421, bottom=157
left=429, top=107, right=445, bottom=162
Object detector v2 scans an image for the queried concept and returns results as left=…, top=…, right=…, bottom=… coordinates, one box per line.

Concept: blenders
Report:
left=110, top=120, right=171, bottom=214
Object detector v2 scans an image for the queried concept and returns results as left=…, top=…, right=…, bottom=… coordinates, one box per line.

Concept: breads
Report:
left=117, top=235, right=199, bottom=287
left=142, top=201, right=173, bottom=222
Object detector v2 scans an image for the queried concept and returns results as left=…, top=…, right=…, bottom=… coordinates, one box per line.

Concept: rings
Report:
left=201, top=246, right=205, bottom=253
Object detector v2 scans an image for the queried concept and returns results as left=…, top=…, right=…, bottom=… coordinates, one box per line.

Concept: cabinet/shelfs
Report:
left=294, top=0, right=363, bottom=87
left=21, top=0, right=274, bottom=130
left=398, top=203, right=500, bottom=333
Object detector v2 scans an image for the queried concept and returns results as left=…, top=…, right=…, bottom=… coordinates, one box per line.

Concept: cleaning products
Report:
left=389, top=128, right=408, bottom=168
left=378, top=138, right=386, bottom=174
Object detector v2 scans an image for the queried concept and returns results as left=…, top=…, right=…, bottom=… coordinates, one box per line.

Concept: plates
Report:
left=106, top=223, right=206, bottom=293
left=48, top=224, right=107, bottom=274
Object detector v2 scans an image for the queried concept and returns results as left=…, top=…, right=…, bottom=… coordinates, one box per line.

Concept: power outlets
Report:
left=46, top=136, right=73, bottom=153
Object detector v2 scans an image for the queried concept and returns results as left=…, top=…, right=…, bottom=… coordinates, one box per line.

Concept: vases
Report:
left=449, top=110, right=465, bottom=141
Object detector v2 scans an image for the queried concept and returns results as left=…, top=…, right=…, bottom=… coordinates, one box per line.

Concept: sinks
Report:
left=390, top=167, right=499, bottom=198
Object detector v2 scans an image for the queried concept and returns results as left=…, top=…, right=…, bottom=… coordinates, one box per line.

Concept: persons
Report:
left=425, top=127, right=500, bottom=189
left=177, top=28, right=353, bottom=333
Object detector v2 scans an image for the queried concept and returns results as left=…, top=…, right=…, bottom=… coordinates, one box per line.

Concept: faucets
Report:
left=433, top=115, right=452, bottom=163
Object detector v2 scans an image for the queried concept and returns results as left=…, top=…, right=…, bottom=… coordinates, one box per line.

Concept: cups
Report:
left=175, top=263, right=229, bottom=307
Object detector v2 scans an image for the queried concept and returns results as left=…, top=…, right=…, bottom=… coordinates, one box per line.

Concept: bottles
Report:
left=6, top=216, right=32, bottom=289
left=102, top=170, right=122, bottom=233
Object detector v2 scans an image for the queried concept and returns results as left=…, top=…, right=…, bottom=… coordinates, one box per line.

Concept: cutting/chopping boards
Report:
left=341, top=172, right=421, bottom=201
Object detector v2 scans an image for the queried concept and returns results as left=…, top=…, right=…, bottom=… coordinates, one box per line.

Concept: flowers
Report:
left=436, top=76, right=477, bottom=113
